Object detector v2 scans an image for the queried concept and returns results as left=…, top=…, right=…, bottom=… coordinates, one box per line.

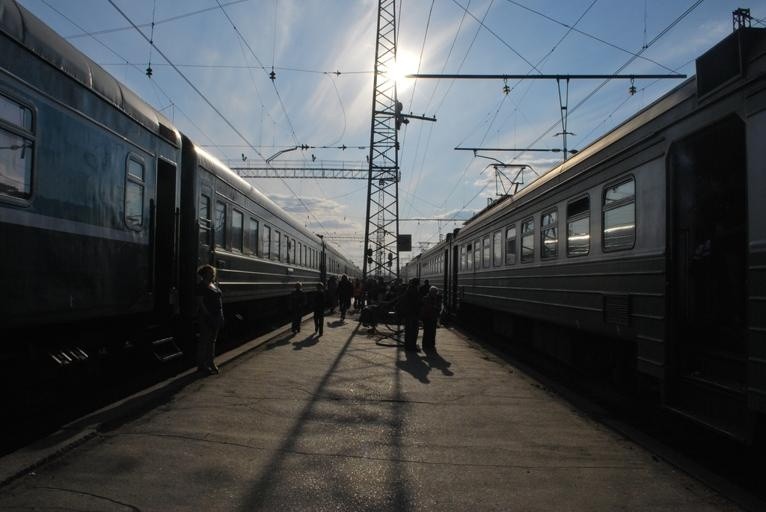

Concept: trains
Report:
left=0, top=0, right=371, bottom=428
left=399, top=29, right=763, bottom=498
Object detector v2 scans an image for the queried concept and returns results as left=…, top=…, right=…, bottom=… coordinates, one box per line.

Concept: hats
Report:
left=198, top=262, right=215, bottom=279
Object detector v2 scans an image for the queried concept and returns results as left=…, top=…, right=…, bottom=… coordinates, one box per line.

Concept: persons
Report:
left=288, top=282, right=304, bottom=332
left=327, top=274, right=443, bottom=351
left=192, top=265, right=223, bottom=379
left=312, top=282, right=326, bottom=336
